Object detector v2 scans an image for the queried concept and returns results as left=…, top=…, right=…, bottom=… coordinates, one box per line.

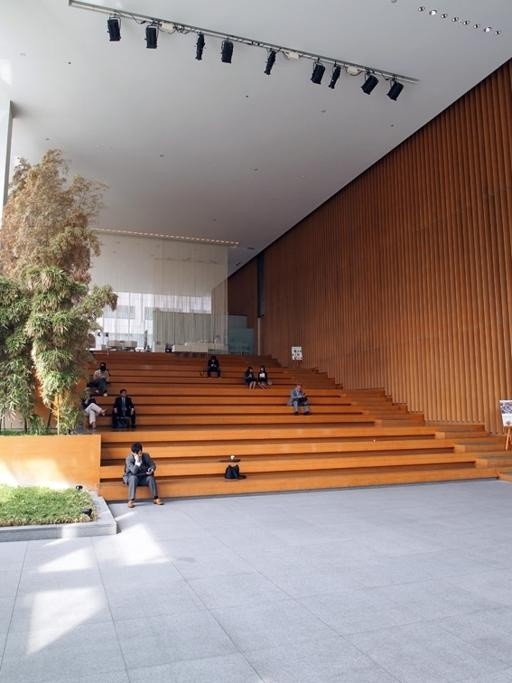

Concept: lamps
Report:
left=65, top=0, right=419, bottom=105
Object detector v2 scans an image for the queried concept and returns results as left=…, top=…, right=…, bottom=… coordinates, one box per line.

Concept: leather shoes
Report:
left=127, top=500, right=135, bottom=508
left=154, top=498, right=164, bottom=505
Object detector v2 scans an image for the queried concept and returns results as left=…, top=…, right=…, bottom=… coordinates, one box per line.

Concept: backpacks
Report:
left=131, top=460, right=154, bottom=478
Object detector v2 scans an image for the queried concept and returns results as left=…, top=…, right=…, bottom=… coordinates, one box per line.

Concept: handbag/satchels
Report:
left=225, top=465, right=247, bottom=479
left=115, top=414, right=131, bottom=429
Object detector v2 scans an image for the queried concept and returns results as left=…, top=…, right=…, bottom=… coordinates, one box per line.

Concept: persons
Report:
left=81, top=386, right=110, bottom=429
left=93, top=361, right=110, bottom=397
left=256, top=364, right=271, bottom=389
left=245, top=365, right=257, bottom=389
left=286, top=383, right=311, bottom=414
left=122, top=441, right=165, bottom=508
left=111, top=387, right=138, bottom=429
left=207, top=354, right=221, bottom=378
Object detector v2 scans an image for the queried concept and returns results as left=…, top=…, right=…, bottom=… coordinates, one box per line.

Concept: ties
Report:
left=123, top=397, right=126, bottom=410
